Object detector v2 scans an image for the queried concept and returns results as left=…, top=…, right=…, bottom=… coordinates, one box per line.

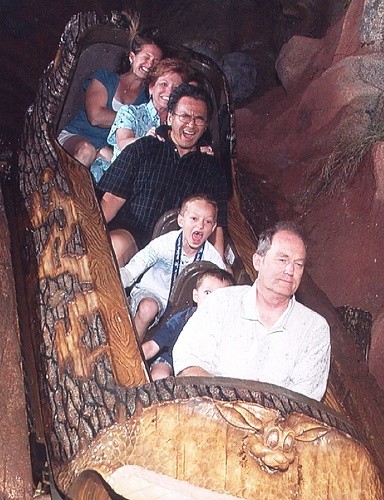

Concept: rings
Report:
left=209, top=147, right=212, bottom=151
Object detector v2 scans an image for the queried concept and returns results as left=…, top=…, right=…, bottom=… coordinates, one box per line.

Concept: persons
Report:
left=107, top=57, right=214, bottom=163
left=140, top=268, right=237, bottom=382
left=173, top=221, right=331, bottom=401
left=96, top=83, right=232, bottom=266
left=57, top=31, right=169, bottom=170
left=119, top=194, right=227, bottom=343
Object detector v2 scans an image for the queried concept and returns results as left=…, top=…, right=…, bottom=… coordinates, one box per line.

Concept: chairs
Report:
left=152, top=208, right=180, bottom=240
left=144, top=261, right=218, bottom=342
